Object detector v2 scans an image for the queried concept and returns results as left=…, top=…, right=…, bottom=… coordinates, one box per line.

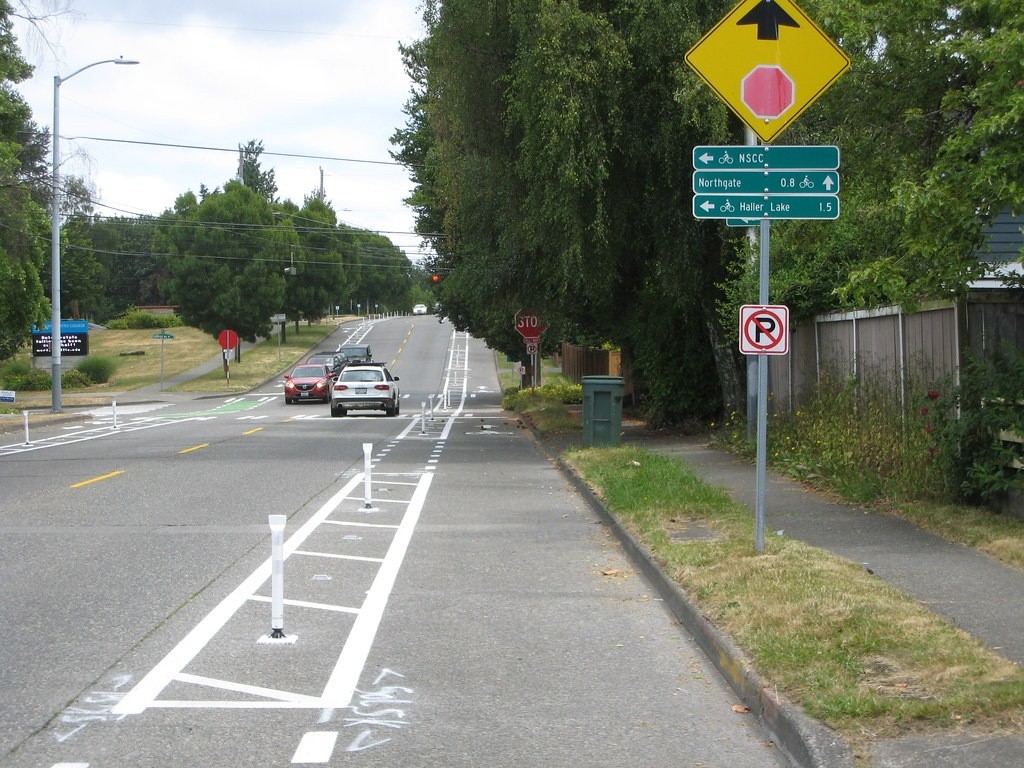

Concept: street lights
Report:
left=51, top=57, right=140, bottom=414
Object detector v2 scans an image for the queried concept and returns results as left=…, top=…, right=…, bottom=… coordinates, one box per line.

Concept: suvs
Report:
left=330, top=362, right=400, bottom=417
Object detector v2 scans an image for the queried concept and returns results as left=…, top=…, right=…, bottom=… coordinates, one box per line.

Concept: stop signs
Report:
left=514, top=307, right=550, bottom=338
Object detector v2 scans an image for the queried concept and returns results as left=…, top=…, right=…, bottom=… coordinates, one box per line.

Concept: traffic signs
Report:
left=692, top=145, right=840, bottom=220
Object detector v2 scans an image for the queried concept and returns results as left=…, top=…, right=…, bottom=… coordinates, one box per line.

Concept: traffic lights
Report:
left=434, top=276, right=439, bottom=281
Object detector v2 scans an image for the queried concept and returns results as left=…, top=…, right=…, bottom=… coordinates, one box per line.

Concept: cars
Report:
left=412, top=304, right=427, bottom=316
left=339, top=344, right=373, bottom=365
left=306, top=352, right=350, bottom=378
left=433, top=302, right=442, bottom=314
left=283, top=364, right=334, bottom=404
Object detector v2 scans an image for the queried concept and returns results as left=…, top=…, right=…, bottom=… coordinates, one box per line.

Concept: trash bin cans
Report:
left=581, top=376, right=626, bottom=449
